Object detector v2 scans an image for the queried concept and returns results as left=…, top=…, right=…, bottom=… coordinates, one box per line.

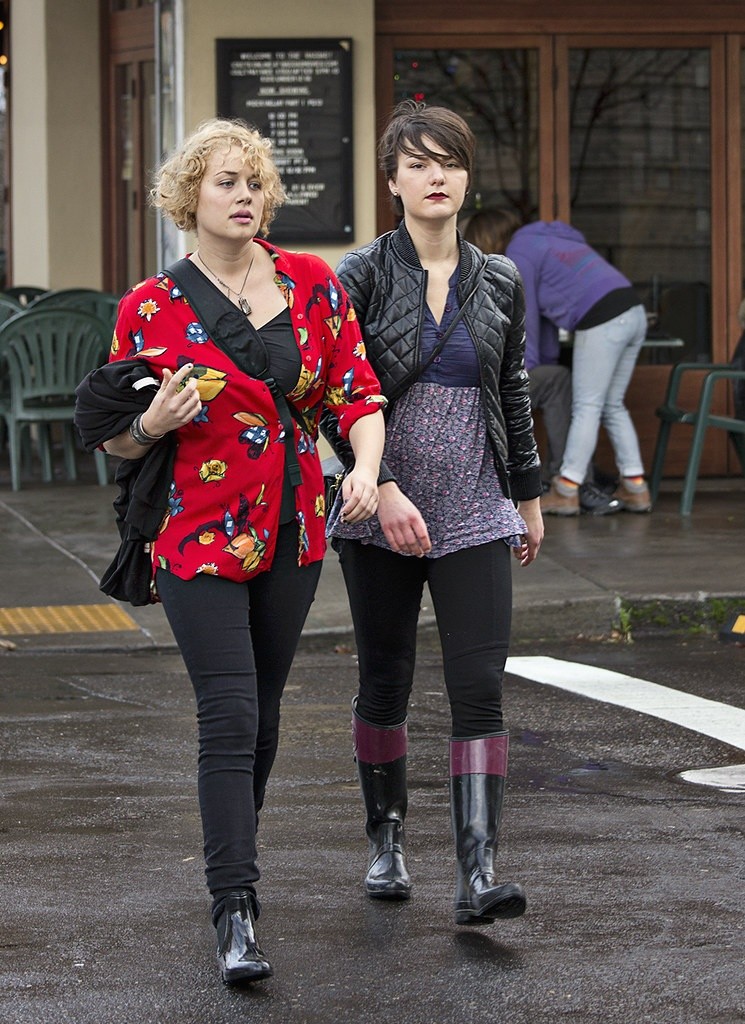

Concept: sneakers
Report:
left=578, top=483, right=623, bottom=513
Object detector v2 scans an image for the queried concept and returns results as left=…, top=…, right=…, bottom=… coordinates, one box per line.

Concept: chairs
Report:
left=648, top=332, right=745, bottom=515
left=0, top=286, right=130, bottom=488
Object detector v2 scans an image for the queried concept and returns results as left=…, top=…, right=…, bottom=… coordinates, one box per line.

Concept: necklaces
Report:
left=197, top=252, right=254, bottom=315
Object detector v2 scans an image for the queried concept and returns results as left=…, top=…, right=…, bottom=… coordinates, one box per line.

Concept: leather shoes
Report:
left=216, top=891, right=272, bottom=983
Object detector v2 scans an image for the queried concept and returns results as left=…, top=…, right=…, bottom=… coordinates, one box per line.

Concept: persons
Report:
left=319, top=97, right=546, bottom=926
left=463, top=205, right=652, bottom=516
left=102, top=116, right=388, bottom=985
left=527, top=362, right=628, bottom=515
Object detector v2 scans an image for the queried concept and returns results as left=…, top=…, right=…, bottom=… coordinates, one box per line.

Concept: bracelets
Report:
left=129, top=413, right=162, bottom=446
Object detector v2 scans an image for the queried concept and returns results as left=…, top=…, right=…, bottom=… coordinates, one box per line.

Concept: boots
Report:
left=351, top=694, right=411, bottom=899
left=448, top=728, right=527, bottom=926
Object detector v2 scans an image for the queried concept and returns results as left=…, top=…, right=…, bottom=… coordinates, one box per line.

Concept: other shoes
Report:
left=606, top=479, right=652, bottom=512
left=538, top=476, right=580, bottom=516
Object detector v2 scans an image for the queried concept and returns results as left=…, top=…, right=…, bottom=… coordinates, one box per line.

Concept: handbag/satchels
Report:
left=326, top=473, right=345, bottom=522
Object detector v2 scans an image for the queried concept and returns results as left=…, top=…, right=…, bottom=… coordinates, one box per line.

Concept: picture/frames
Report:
left=214, top=35, right=353, bottom=247
